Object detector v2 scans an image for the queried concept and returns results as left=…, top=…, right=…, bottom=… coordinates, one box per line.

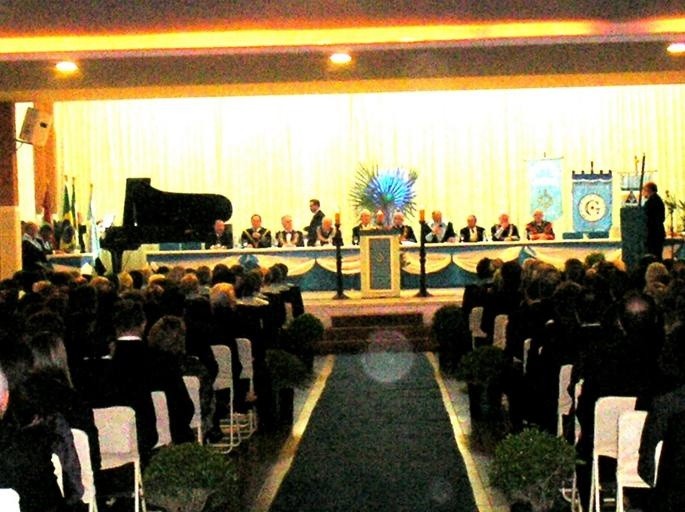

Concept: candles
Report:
left=419, top=207, right=424, bottom=221
left=334, top=209, right=340, bottom=224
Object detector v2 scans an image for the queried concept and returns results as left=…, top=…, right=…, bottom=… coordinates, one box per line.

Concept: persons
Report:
left=21, top=219, right=74, bottom=269
left=641, top=183, right=666, bottom=262
left=206, top=219, right=232, bottom=250
left=274, top=215, right=304, bottom=247
left=1, top=261, right=303, bottom=510
left=491, top=213, right=521, bottom=241
left=352, top=209, right=486, bottom=245
left=241, top=215, right=272, bottom=248
left=303, top=200, right=325, bottom=246
left=526, top=209, right=555, bottom=240
left=312, top=216, right=343, bottom=246
left=449, top=251, right=684, bottom=510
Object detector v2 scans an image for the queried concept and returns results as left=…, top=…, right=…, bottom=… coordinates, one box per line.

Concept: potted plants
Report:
left=428, top=302, right=468, bottom=366
left=287, top=310, right=323, bottom=368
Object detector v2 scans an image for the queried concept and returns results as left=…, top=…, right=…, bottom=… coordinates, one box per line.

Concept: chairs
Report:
left=562, top=230, right=609, bottom=239
left=470, top=307, right=663, bottom=512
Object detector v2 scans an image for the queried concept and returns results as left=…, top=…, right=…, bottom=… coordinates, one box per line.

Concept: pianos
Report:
left=99, top=178, right=232, bottom=277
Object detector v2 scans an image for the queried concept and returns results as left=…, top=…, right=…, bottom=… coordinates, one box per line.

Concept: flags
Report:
left=64, top=184, right=101, bottom=264
left=572, top=174, right=613, bottom=232
left=526, top=159, right=563, bottom=222
